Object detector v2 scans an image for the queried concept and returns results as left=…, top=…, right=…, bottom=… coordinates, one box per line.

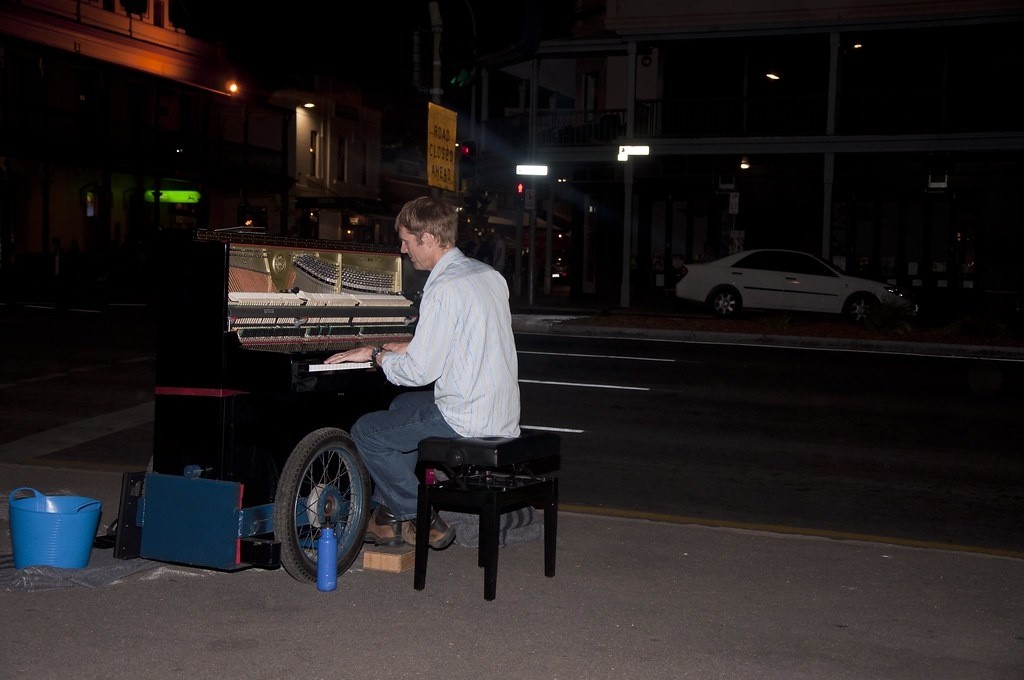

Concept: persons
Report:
left=466, top=231, right=506, bottom=272
left=324, top=195, right=521, bottom=548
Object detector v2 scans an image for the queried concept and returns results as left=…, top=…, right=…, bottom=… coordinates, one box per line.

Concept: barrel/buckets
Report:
left=9, top=486, right=102, bottom=571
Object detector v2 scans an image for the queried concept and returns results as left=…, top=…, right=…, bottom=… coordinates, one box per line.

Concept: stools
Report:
left=409, top=428, right=561, bottom=600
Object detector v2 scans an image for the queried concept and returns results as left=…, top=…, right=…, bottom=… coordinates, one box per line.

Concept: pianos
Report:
left=152, top=226, right=424, bottom=569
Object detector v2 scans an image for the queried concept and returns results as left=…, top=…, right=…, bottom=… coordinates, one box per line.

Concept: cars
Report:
left=670, top=248, right=914, bottom=336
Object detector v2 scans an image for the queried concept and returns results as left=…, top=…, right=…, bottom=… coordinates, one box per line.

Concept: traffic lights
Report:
left=460, top=139, right=476, bottom=180
left=515, top=181, right=525, bottom=198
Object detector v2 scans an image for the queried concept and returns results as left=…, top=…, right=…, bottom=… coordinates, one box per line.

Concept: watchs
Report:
left=371, top=347, right=386, bottom=367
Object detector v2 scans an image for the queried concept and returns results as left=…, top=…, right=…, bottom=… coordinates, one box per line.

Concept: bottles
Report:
left=317, top=516, right=339, bottom=591
left=86, top=186, right=95, bottom=216
left=175, top=203, right=196, bottom=228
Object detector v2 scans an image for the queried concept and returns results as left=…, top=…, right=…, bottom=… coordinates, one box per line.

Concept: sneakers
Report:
left=363, top=507, right=403, bottom=548
left=402, top=512, right=456, bottom=549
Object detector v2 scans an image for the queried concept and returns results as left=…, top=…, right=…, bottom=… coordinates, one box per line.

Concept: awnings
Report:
left=485, top=210, right=559, bottom=230
